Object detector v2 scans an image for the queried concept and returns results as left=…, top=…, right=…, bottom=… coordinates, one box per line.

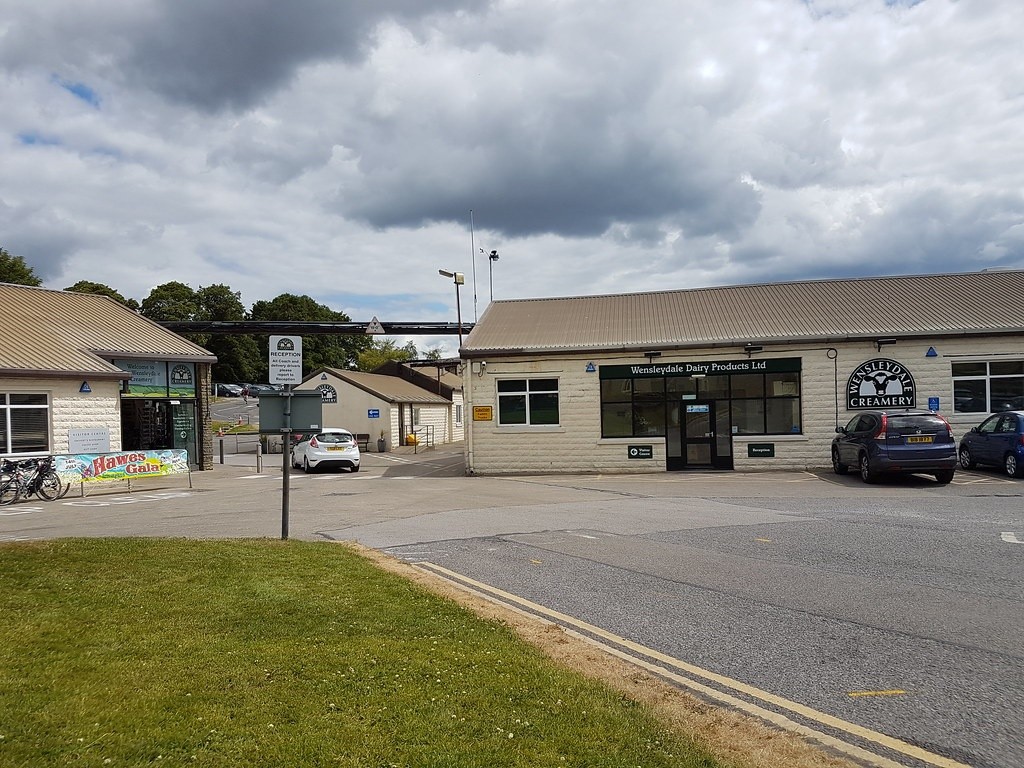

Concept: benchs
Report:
left=352, top=433, right=370, bottom=452
left=278, top=435, right=297, bottom=453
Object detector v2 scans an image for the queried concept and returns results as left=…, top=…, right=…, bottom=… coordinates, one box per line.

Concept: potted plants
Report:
left=259, top=435, right=270, bottom=454
left=377, top=428, right=387, bottom=452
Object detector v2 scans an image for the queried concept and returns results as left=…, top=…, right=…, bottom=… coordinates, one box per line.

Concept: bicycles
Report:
left=0, top=456, right=62, bottom=506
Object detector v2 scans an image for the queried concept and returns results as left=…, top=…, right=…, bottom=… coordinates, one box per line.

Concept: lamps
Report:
left=744, top=343, right=763, bottom=358
left=876, top=338, right=898, bottom=353
left=644, top=351, right=662, bottom=362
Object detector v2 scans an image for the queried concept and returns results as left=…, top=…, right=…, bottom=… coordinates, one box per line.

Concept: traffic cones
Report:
left=237, top=415, right=243, bottom=427
left=216, top=425, right=225, bottom=438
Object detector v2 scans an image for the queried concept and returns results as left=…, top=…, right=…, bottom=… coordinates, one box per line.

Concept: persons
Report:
left=242, top=388, right=249, bottom=402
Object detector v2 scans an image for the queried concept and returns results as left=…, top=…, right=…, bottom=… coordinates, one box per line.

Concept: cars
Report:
left=958, top=408, right=1024, bottom=477
left=831, top=407, right=959, bottom=484
left=292, top=427, right=360, bottom=473
left=210, top=382, right=284, bottom=399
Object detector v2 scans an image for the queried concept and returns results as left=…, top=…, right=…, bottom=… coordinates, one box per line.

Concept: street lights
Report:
left=480, top=247, right=500, bottom=303
left=437, top=268, right=463, bottom=372
